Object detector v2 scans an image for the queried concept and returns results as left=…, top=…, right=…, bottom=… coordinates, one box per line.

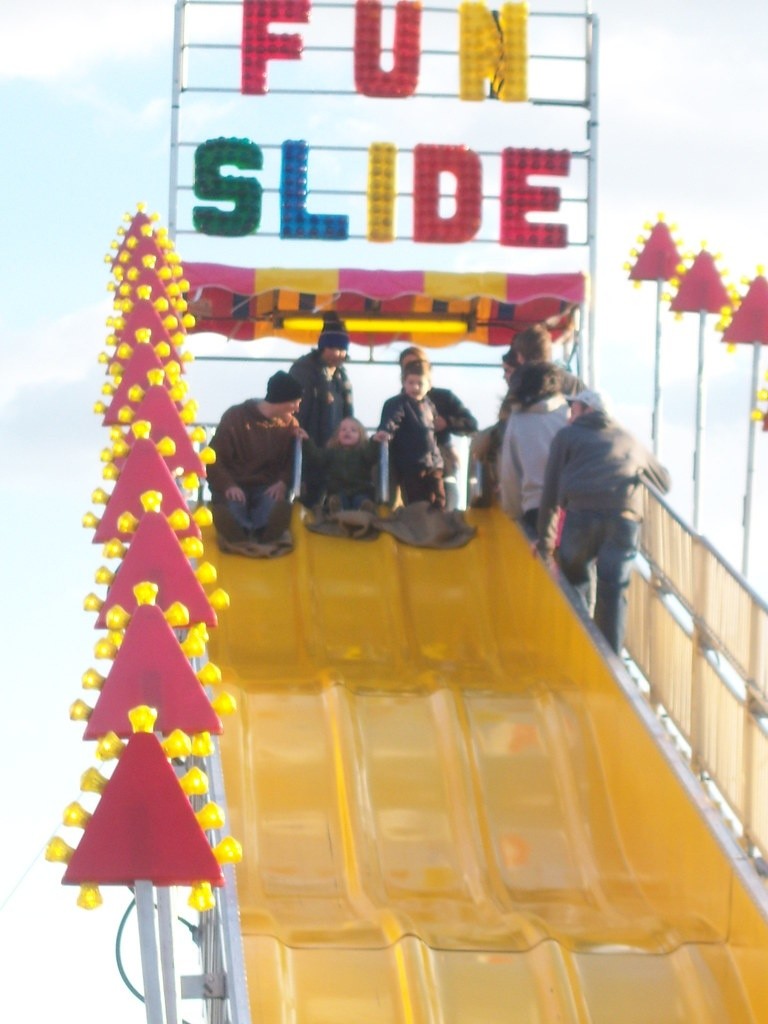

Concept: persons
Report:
left=288, top=311, right=354, bottom=447
left=378, top=346, right=478, bottom=513
left=207, top=369, right=303, bottom=545
left=472, top=324, right=670, bottom=658
left=299, top=416, right=389, bottom=515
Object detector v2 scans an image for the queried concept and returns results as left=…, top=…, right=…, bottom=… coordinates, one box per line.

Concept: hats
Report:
left=566, top=390, right=604, bottom=411
left=319, top=311, right=349, bottom=347
left=265, top=371, right=304, bottom=403
